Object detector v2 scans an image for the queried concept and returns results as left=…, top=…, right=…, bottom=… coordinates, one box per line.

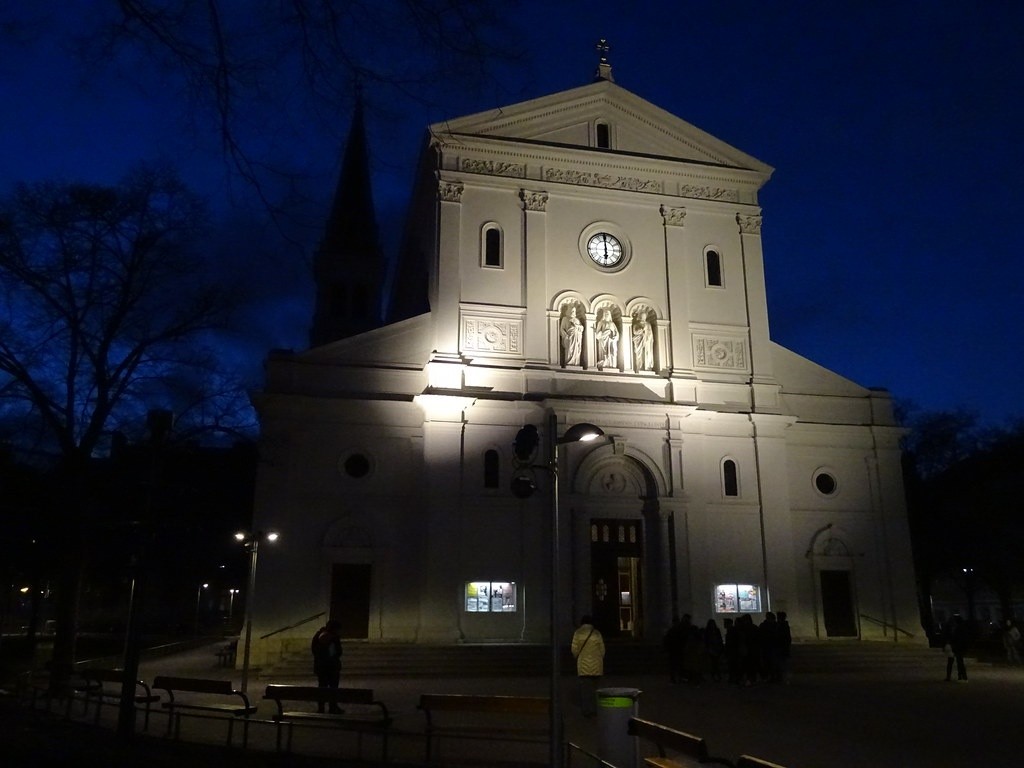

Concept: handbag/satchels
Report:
left=1013, top=639, right=1024, bottom=651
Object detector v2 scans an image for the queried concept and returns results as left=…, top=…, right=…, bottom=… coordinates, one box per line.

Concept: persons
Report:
left=573, top=614, right=605, bottom=717
left=560, top=306, right=583, bottom=366
left=941, top=607, right=968, bottom=684
left=311, top=620, right=345, bottom=714
left=632, top=310, right=655, bottom=372
left=664, top=611, right=792, bottom=693
left=1002, top=618, right=1021, bottom=667
left=597, top=309, right=620, bottom=368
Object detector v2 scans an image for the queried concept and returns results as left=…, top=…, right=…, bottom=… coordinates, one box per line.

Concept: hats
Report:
left=765, top=612, right=776, bottom=621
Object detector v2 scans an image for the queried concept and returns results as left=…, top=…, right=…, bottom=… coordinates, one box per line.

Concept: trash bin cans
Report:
left=595, top=687, right=641, bottom=768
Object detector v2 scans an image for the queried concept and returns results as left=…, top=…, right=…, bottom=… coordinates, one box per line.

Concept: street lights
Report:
left=515, top=414, right=566, bottom=768
left=231, top=530, right=281, bottom=692
left=18, top=584, right=50, bottom=663
left=193, top=583, right=209, bottom=639
left=228, top=588, right=235, bottom=629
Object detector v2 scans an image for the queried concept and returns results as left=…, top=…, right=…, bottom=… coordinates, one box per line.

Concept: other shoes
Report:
left=328, top=705, right=344, bottom=714
left=316, top=707, right=324, bottom=713
left=583, top=711, right=596, bottom=718
left=955, top=680, right=968, bottom=684
